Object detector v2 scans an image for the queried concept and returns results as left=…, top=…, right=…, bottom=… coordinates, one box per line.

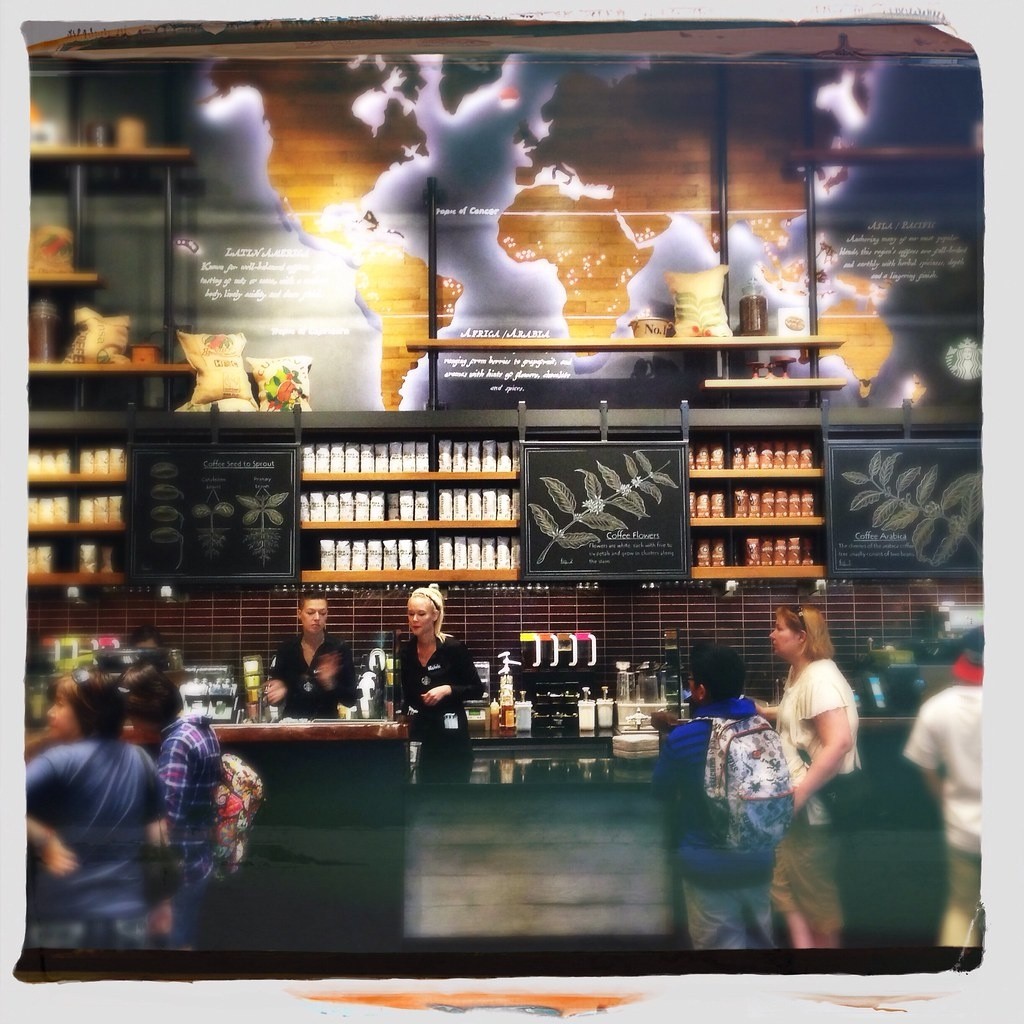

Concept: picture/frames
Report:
left=125, top=441, right=301, bottom=586
left=824, top=439, right=983, bottom=579
left=519, top=439, right=692, bottom=582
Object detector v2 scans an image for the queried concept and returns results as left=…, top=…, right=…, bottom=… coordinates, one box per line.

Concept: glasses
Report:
left=71, top=666, right=96, bottom=704
left=790, top=605, right=806, bottom=630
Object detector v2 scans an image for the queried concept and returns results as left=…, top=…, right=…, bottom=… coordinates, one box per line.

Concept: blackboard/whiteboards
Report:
left=520, top=441, right=692, bottom=580
left=824, top=439, right=982, bottom=578
left=124, top=443, right=300, bottom=586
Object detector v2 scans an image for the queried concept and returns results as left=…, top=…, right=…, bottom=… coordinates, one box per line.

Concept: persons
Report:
left=24, top=668, right=173, bottom=949
left=118, top=664, right=222, bottom=948
left=902, top=625, right=983, bottom=946
left=654, top=640, right=778, bottom=949
left=262, top=593, right=358, bottom=718
left=400, top=585, right=485, bottom=782
left=754, top=608, right=862, bottom=948
left=128, top=623, right=163, bottom=648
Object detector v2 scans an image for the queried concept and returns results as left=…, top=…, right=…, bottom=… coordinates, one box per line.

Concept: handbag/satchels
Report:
left=685, top=713, right=794, bottom=859
left=206, top=751, right=266, bottom=881
left=818, top=766, right=866, bottom=834
left=135, top=745, right=176, bottom=905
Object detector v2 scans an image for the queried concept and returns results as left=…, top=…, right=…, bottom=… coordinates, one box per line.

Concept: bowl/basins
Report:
left=628, top=317, right=670, bottom=338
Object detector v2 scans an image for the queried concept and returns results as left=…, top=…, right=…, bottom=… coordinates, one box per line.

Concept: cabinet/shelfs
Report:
left=28, top=145, right=844, bottom=586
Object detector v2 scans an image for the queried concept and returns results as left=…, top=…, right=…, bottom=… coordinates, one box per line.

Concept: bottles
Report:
left=738, top=277, right=767, bottom=335
left=490, top=697, right=499, bottom=730
left=501, top=696, right=516, bottom=731
left=28, top=296, right=60, bottom=364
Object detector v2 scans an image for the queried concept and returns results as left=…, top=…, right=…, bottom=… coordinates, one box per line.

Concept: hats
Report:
left=952, top=625, right=984, bottom=685
